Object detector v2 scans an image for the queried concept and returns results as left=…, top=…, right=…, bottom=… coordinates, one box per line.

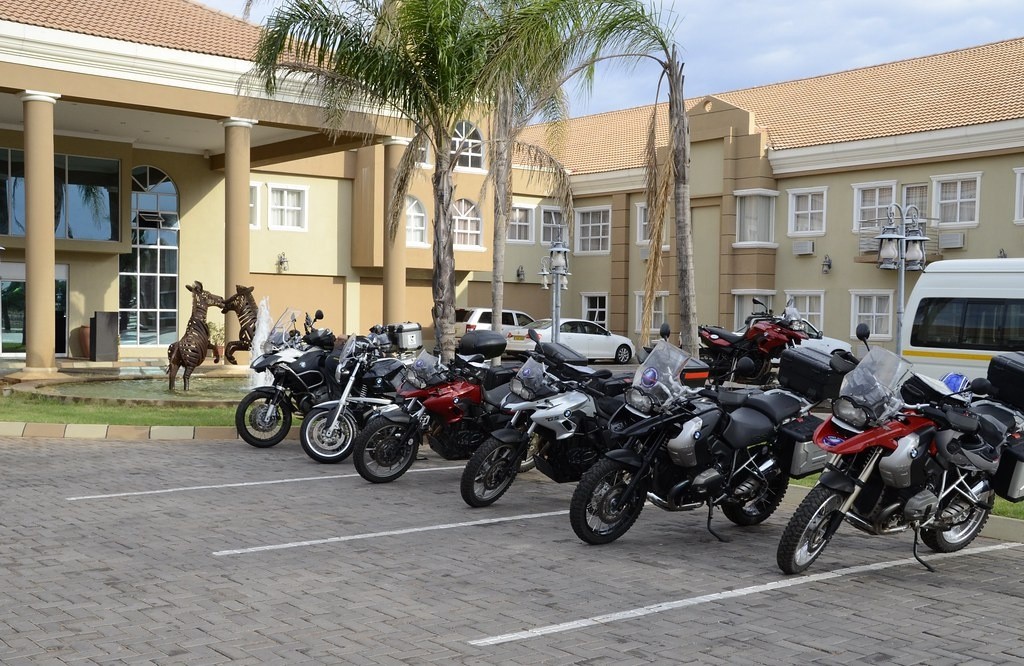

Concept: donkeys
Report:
left=164, top=281, right=259, bottom=391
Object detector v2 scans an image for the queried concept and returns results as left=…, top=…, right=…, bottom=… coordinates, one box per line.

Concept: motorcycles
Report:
left=352, top=329, right=524, bottom=485
left=568, top=343, right=840, bottom=547
left=698, top=298, right=820, bottom=384
left=777, top=352, right=1024, bottom=575
left=300, top=336, right=482, bottom=465
left=234, top=310, right=369, bottom=449
left=459, top=339, right=678, bottom=511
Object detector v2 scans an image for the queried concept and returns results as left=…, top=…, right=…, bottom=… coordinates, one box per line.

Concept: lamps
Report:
left=820, top=254, right=833, bottom=275
left=517, top=265, right=525, bottom=282
left=279, top=252, right=289, bottom=271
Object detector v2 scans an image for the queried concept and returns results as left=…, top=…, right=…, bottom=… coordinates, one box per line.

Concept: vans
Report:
left=896, top=255, right=1023, bottom=418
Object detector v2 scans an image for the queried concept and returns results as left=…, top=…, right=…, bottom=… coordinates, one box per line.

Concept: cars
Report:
left=504, top=316, right=636, bottom=364
left=456, top=307, right=538, bottom=343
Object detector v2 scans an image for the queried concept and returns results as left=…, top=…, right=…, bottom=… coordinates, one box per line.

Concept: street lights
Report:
left=873, top=201, right=928, bottom=357
left=540, top=236, right=572, bottom=346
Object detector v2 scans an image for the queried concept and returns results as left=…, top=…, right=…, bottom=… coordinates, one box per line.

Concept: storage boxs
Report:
left=388, top=322, right=423, bottom=350
left=995, top=443, right=1024, bottom=503
left=459, top=330, right=507, bottom=360
left=679, top=357, right=710, bottom=389
left=778, top=347, right=845, bottom=402
left=535, top=342, right=588, bottom=381
left=773, top=414, right=834, bottom=480
left=986, top=351, right=1024, bottom=397
left=484, top=363, right=523, bottom=391
